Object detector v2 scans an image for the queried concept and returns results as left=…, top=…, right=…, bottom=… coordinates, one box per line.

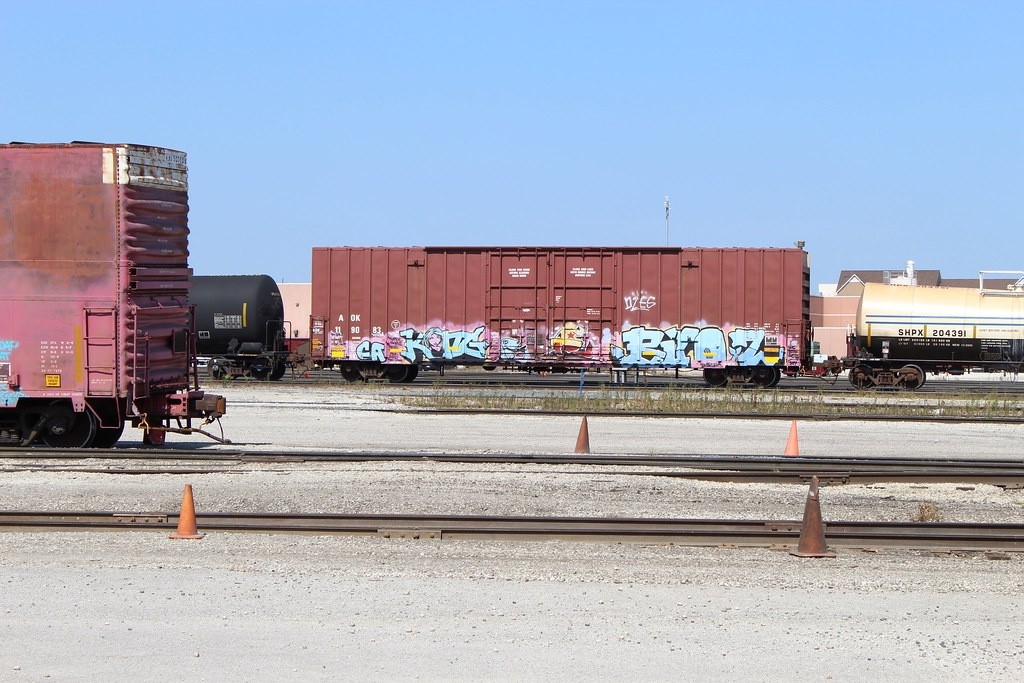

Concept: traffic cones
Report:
left=574, top=416, right=590, bottom=454
left=789, top=475, right=837, bottom=559
left=782, top=419, right=799, bottom=457
left=167, top=483, right=206, bottom=539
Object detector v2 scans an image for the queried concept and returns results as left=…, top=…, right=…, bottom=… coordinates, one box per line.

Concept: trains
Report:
left=0, top=142, right=227, bottom=450
left=192, top=243, right=1024, bottom=391
